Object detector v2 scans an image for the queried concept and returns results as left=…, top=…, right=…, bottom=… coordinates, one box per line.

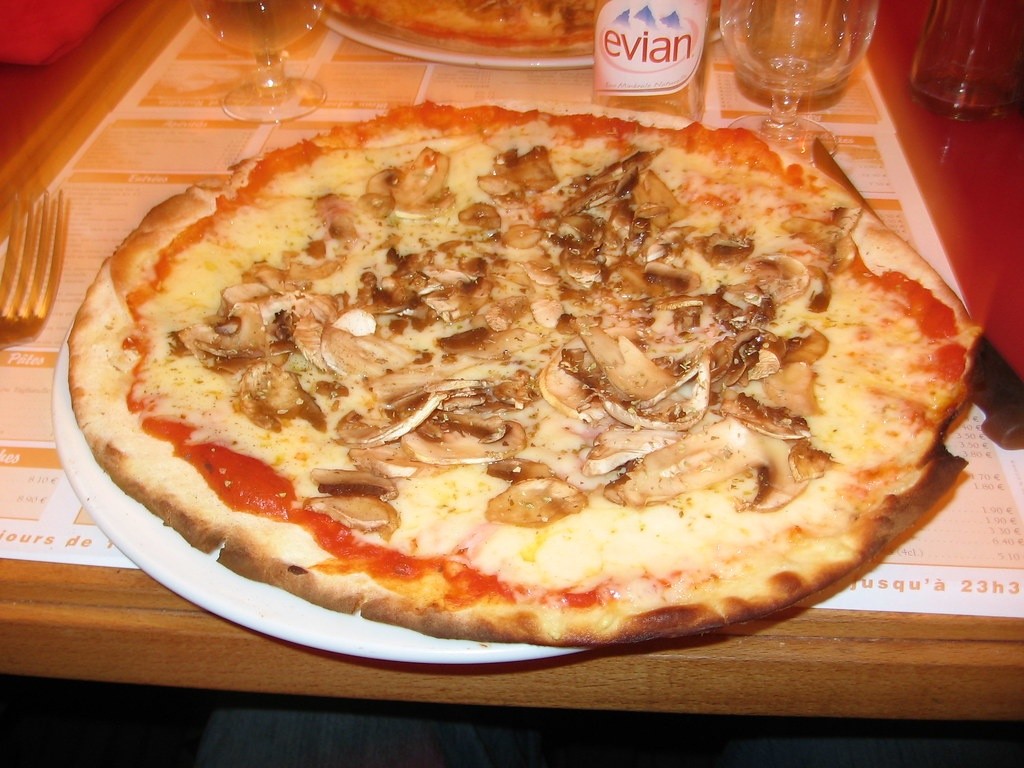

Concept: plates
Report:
left=314, top=7, right=724, bottom=71
left=51, top=313, right=622, bottom=663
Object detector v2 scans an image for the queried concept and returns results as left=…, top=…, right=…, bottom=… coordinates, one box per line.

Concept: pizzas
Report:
left=322, top=0, right=735, bottom=56
left=64, top=101, right=984, bottom=646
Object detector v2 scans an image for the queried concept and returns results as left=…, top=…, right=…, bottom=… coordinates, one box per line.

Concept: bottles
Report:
left=591, top=0, right=710, bottom=122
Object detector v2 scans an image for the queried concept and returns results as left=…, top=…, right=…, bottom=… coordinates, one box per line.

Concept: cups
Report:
left=907, top=0, right=1024, bottom=123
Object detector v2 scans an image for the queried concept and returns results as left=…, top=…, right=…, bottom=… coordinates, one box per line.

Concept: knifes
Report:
left=813, top=137, right=1024, bottom=452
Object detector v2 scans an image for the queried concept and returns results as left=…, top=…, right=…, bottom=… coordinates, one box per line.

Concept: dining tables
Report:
left=0, top=0, right=1024, bottom=722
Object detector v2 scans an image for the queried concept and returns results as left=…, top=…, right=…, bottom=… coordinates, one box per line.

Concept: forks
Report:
left=0, top=189, right=69, bottom=351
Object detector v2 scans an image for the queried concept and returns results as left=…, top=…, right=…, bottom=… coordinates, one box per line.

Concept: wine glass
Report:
left=189, top=0, right=327, bottom=124
left=715, top=0, right=882, bottom=170
